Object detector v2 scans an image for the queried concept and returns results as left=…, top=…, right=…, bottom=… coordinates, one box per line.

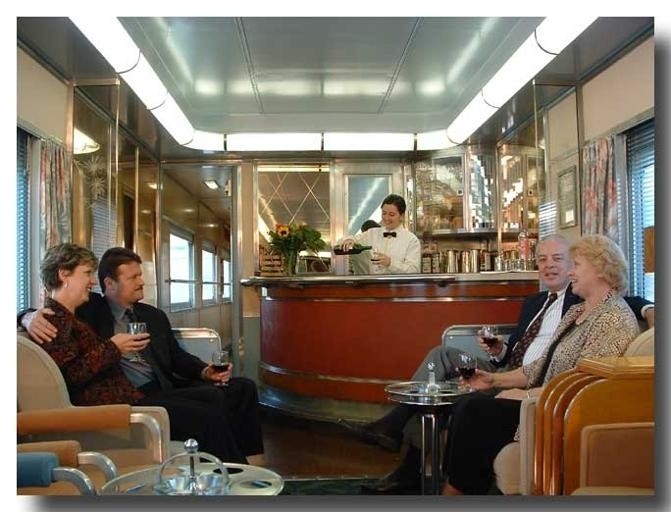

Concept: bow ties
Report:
left=383, top=233, right=396, bottom=237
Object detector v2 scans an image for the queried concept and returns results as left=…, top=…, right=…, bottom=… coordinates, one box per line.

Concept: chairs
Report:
left=17, top=405, right=162, bottom=495
left=17, top=453, right=98, bottom=495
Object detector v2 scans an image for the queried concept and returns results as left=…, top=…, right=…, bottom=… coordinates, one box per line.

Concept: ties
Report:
left=125, top=308, right=175, bottom=391
left=508, top=293, right=558, bottom=370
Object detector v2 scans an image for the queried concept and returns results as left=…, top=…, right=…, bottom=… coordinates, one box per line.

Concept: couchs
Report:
left=18, top=327, right=221, bottom=464
left=441, top=324, right=655, bottom=495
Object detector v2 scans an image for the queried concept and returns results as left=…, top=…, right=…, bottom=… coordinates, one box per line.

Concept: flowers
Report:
left=269, top=223, right=327, bottom=275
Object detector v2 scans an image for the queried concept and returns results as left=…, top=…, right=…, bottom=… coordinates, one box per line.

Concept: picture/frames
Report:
left=557, top=164, right=578, bottom=229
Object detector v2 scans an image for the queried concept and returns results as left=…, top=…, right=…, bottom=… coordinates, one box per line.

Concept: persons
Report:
left=16, top=246, right=265, bottom=457
left=339, top=235, right=654, bottom=494
left=28, top=243, right=249, bottom=474
left=348, top=219, right=381, bottom=275
left=444, top=235, right=641, bottom=495
left=338, top=194, right=422, bottom=274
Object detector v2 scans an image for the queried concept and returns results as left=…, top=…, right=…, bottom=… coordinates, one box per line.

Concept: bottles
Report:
left=422, top=241, right=431, bottom=273
left=480, top=243, right=488, bottom=271
left=431, top=240, right=440, bottom=274
left=471, top=249, right=479, bottom=272
left=461, top=251, right=470, bottom=273
left=334, top=244, right=371, bottom=255
left=445, top=249, right=459, bottom=273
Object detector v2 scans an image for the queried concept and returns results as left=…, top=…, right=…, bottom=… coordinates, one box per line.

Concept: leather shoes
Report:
left=339, top=417, right=402, bottom=452
left=361, top=467, right=421, bottom=493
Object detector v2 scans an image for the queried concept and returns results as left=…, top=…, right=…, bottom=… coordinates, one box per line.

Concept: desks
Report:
left=385, top=380, right=479, bottom=494
left=98, top=438, right=284, bottom=496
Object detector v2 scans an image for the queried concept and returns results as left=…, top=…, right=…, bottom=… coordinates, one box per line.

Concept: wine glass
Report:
left=212, top=351, right=230, bottom=387
left=481, top=326, right=498, bottom=358
left=369, top=248, right=384, bottom=270
left=458, top=352, right=477, bottom=393
left=129, top=322, right=147, bottom=363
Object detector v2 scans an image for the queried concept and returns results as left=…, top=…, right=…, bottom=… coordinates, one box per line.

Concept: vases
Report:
left=288, top=247, right=299, bottom=275
